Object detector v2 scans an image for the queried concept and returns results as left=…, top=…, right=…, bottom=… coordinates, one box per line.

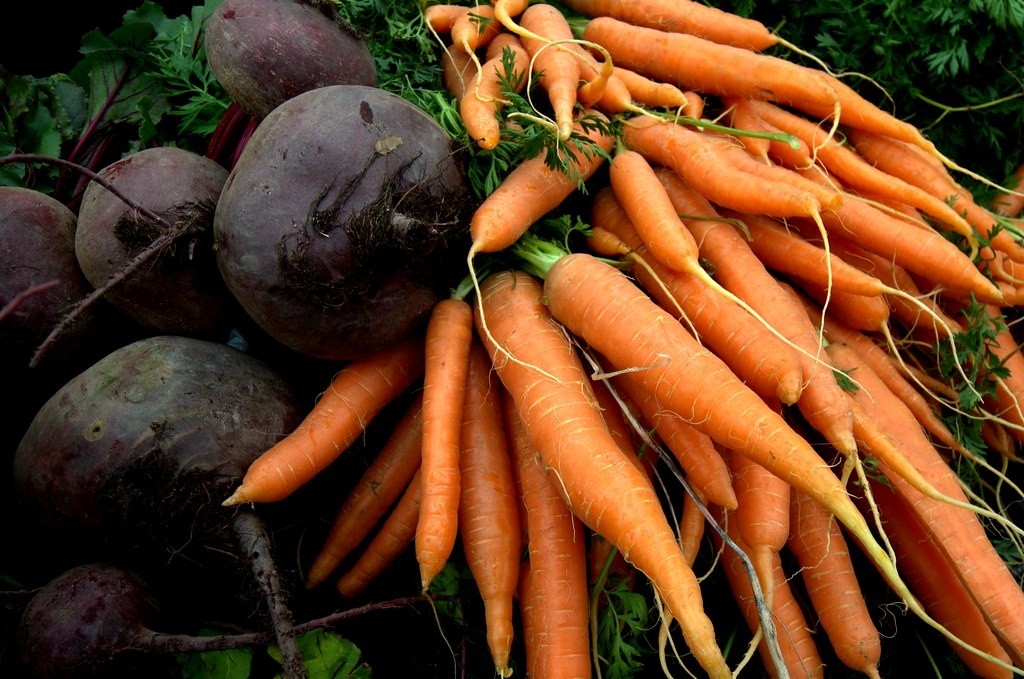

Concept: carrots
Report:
left=0, top=0, right=1024, bottom=679
